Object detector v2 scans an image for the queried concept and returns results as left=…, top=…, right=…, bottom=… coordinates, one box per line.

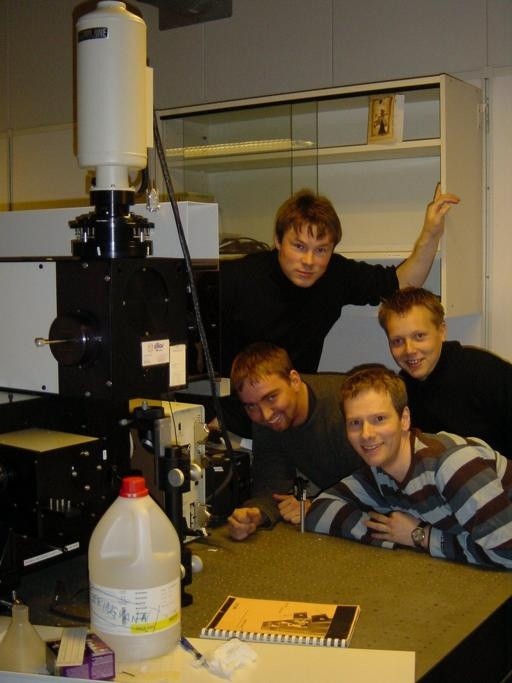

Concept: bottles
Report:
left=2, top=606, right=46, bottom=674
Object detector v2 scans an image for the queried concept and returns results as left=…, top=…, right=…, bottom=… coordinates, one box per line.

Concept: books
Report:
left=200, top=594, right=361, bottom=648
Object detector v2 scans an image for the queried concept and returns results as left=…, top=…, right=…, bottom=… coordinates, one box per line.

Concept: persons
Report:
left=377, top=284, right=512, bottom=459
left=198, top=181, right=461, bottom=375
left=303, top=362, right=512, bottom=573
left=225, top=344, right=367, bottom=541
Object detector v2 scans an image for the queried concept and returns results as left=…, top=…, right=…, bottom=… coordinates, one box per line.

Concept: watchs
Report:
left=411, top=520, right=428, bottom=552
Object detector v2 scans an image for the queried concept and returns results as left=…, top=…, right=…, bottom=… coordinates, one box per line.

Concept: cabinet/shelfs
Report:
left=149, top=73, right=483, bottom=320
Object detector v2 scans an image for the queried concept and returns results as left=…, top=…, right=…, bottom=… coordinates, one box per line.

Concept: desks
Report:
left=49, top=514, right=512, bottom=683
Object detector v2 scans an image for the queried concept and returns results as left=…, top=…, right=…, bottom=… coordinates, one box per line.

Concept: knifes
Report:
left=181, top=637, right=210, bottom=669
